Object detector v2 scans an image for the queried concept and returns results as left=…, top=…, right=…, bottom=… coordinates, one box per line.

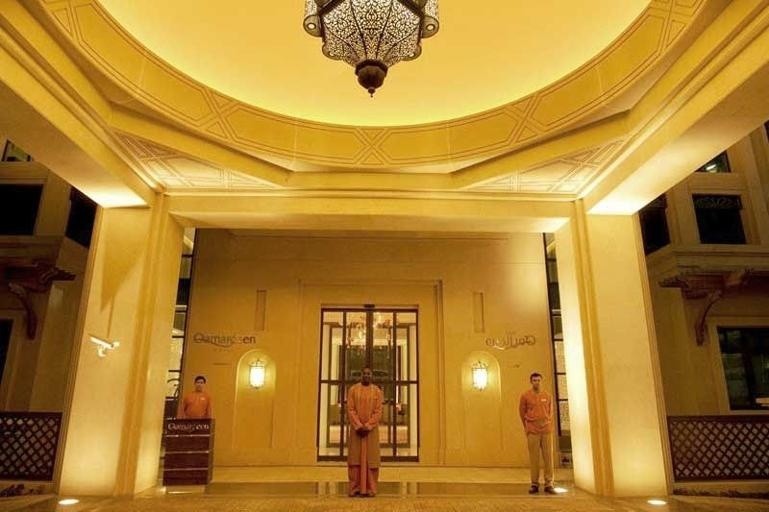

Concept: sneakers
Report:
left=545, top=487, right=556, bottom=494
left=529, top=486, right=538, bottom=493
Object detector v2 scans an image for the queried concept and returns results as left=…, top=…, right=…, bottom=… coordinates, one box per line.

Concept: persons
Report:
left=177, top=376, right=212, bottom=418
left=348, top=368, right=383, bottom=497
left=520, top=373, right=556, bottom=494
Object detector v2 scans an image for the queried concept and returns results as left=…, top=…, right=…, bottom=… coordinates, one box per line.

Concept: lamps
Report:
left=300, top=1, right=441, bottom=98
